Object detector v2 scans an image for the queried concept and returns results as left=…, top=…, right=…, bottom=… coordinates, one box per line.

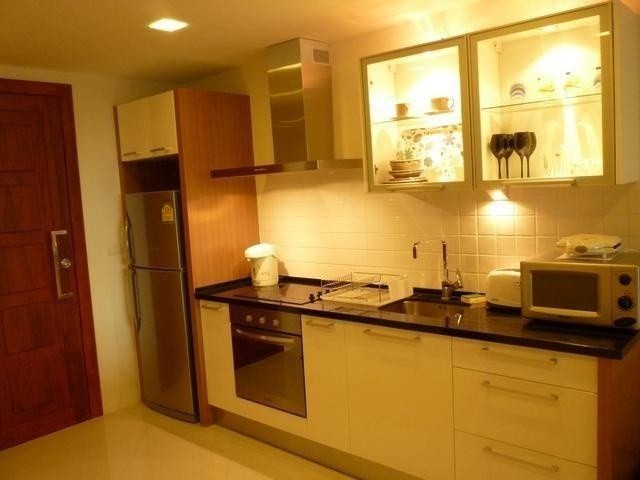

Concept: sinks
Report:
left=377, top=297, right=472, bottom=318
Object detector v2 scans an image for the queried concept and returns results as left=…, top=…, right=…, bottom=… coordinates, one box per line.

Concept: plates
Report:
left=381, top=178, right=427, bottom=185
left=386, top=168, right=423, bottom=178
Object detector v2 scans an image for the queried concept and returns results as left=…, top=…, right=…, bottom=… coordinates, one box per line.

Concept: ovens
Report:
left=230, top=303, right=307, bottom=419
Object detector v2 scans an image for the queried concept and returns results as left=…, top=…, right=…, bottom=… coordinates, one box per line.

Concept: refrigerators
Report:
left=123, top=190, right=198, bottom=423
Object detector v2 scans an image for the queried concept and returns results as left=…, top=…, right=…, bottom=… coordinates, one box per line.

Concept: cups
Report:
left=431, top=97, right=454, bottom=111
left=395, top=103, right=408, bottom=117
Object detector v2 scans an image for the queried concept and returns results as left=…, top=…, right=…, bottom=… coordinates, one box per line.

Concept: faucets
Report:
left=412, top=238, right=464, bottom=300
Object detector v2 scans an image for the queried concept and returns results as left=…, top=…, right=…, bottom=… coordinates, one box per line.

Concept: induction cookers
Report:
left=195, top=268, right=350, bottom=306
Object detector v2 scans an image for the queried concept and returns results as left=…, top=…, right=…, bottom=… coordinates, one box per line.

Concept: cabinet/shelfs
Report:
left=199, top=299, right=237, bottom=433
left=300, top=315, right=350, bottom=473
left=112, top=87, right=261, bottom=424
left=344, top=320, right=452, bottom=479
left=357, top=0, right=640, bottom=195
left=451, top=335, right=640, bottom=480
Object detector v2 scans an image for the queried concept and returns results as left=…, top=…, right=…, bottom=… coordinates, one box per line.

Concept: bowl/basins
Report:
left=381, top=158, right=429, bottom=170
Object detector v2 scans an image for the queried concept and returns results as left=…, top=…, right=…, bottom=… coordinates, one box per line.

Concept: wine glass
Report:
left=490, top=134, right=508, bottom=179
left=504, top=134, right=514, bottom=178
left=514, top=133, right=531, bottom=178
left=525, top=132, right=537, bottom=178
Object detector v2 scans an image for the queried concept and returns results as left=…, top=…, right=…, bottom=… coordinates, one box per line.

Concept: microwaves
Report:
left=521, top=246, right=640, bottom=329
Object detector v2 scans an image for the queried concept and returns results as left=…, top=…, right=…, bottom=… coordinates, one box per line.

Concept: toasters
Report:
left=485, top=267, right=521, bottom=314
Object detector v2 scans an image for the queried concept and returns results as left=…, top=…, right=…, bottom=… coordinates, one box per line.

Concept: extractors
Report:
left=210, top=39, right=363, bottom=179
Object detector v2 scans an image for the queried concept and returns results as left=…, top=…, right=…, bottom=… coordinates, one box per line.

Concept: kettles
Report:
left=243, top=243, right=280, bottom=287
